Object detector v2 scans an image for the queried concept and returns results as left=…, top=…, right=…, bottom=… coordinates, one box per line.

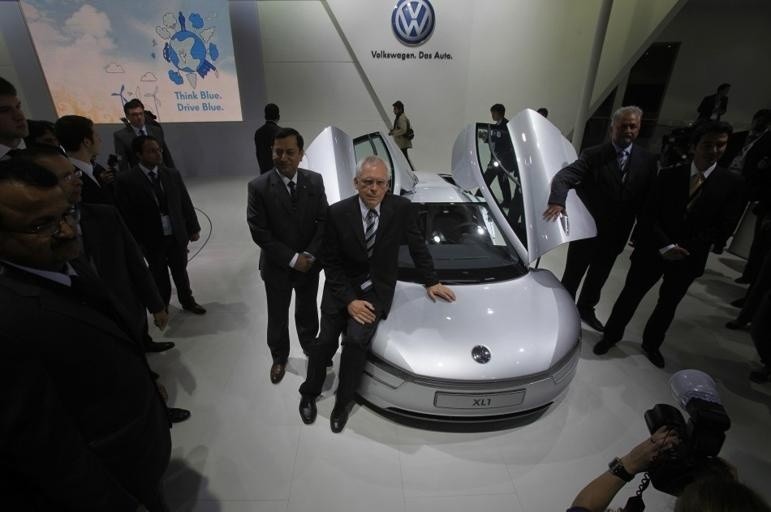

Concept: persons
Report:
left=388, top=100, right=416, bottom=172
left=475, top=102, right=518, bottom=207
left=246, top=127, right=335, bottom=386
left=692, top=82, right=733, bottom=127
left=590, top=120, right=741, bottom=370
left=543, top=104, right=657, bottom=334
left=255, top=102, right=283, bottom=175
left=2, top=77, right=209, bottom=508
left=565, top=422, right=770, bottom=511
left=537, top=107, right=547, bottom=118
left=298, top=155, right=456, bottom=431
left=710, top=107, right=771, bottom=385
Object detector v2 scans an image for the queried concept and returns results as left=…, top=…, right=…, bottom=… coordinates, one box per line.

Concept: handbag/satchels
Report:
left=406, top=128, right=414, bottom=140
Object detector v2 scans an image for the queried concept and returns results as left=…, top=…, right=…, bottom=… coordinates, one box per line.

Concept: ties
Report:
left=288, top=182, right=297, bottom=206
left=139, top=129, right=145, bottom=135
left=70, top=275, right=123, bottom=326
left=618, top=152, right=628, bottom=176
left=357, top=210, right=377, bottom=289
left=148, top=172, right=167, bottom=216
left=93, top=167, right=103, bottom=187
left=689, top=172, right=701, bottom=198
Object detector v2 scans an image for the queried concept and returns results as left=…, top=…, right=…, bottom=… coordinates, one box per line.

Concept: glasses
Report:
left=65, top=170, right=83, bottom=182
left=10, top=206, right=81, bottom=233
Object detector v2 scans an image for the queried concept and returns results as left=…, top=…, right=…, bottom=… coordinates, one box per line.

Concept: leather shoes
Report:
left=144, top=342, right=174, bottom=352
left=751, top=365, right=770, bottom=384
left=299, top=397, right=316, bottom=424
left=587, top=317, right=604, bottom=331
left=164, top=408, right=189, bottom=421
left=330, top=406, right=347, bottom=433
left=642, top=343, right=664, bottom=368
left=711, top=242, right=726, bottom=254
left=189, top=301, right=206, bottom=314
left=732, top=297, right=745, bottom=308
left=733, top=276, right=750, bottom=290
left=726, top=317, right=746, bottom=330
left=270, top=363, right=284, bottom=383
left=594, top=337, right=616, bottom=355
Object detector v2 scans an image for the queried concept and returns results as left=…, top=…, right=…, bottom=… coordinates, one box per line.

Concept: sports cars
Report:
left=295, top=106, right=599, bottom=427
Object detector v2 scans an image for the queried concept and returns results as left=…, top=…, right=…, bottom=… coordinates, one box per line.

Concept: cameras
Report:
left=644, top=370, right=731, bottom=495
left=107, top=152, right=121, bottom=181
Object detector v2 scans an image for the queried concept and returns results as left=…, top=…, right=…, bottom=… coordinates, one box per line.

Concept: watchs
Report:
left=609, top=456, right=635, bottom=483
